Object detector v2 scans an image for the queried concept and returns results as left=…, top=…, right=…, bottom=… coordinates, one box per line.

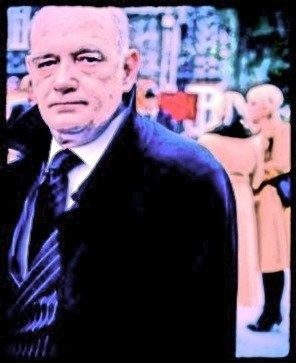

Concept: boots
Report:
left=245, top=270, right=284, bottom=332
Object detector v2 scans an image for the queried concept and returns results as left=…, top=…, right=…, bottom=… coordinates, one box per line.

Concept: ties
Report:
left=26, top=146, right=84, bottom=276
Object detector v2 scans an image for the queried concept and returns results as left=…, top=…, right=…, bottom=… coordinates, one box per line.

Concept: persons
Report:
left=245, top=83, right=290, bottom=333
left=201, top=91, right=264, bottom=318
left=5, top=6, right=236, bottom=357
